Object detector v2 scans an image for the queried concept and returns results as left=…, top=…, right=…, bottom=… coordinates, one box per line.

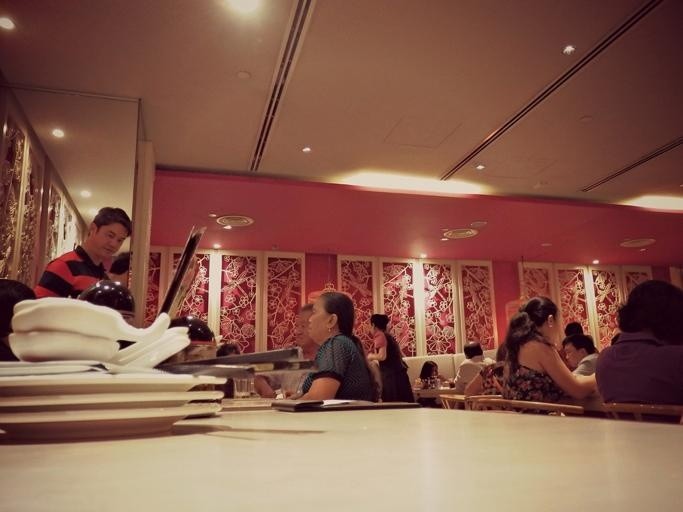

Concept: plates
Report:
left=1, top=361, right=231, bottom=442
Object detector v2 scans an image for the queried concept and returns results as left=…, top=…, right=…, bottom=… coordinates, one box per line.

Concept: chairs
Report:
left=440, top=392, right=681, bottom=425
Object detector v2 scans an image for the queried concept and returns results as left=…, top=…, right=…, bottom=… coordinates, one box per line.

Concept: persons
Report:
left=213, top=344, right=241, bottom=399
left=453, top=279, right=683, bottom=425
left=253, top=303, right=321, bottom=399
left=366, top=313, right=416, bottom=403
left=101, top=252, right=130, bottom=288
left=282, top=291, right=375, bottom=402
left=33, top=206, right=132, bottom=300
left=412, top=361, right=449, bottom=409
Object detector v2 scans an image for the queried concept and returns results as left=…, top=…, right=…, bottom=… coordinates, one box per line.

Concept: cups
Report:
left=232, top=377, right=254, bottom=399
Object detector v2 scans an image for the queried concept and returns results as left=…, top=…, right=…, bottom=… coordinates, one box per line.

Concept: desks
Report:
left=1, top=398, right=680, bottom=511
left=1, top=398, right=682, bottom=511
